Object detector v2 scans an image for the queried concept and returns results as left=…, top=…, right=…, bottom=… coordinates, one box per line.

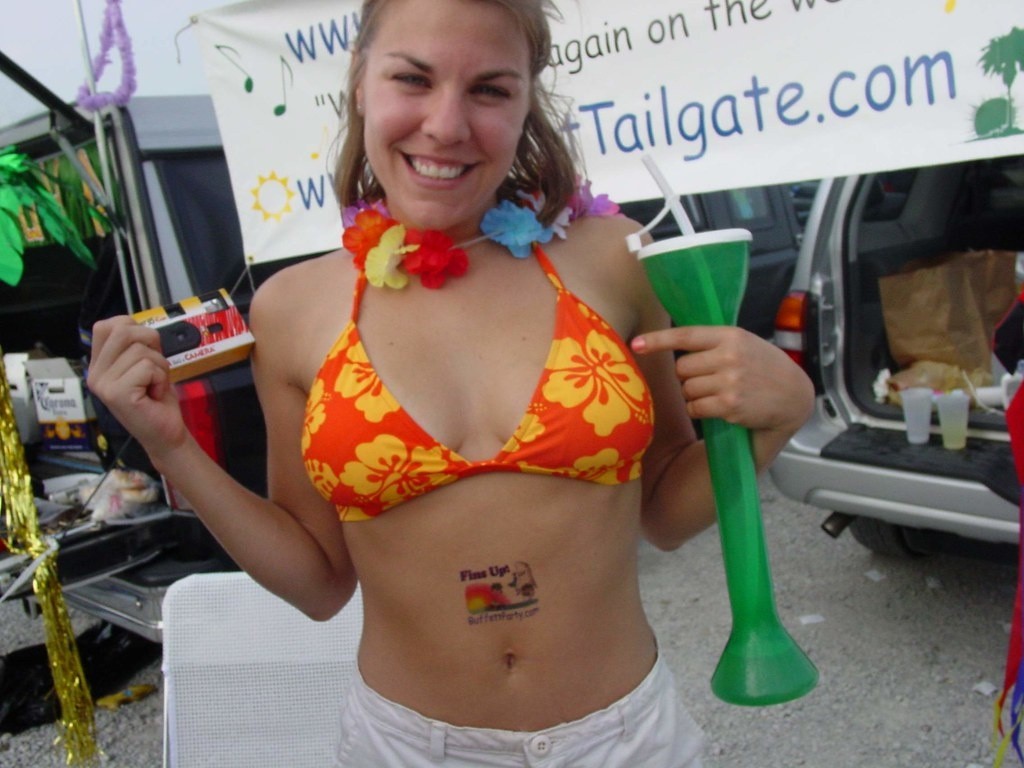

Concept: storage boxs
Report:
left=25, top=357, right=97, bottom=451
left=4, top=352, right=53, bottom=446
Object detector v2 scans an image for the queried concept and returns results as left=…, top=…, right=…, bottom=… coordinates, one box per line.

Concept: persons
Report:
left=87, top=0, right=815, bottom=768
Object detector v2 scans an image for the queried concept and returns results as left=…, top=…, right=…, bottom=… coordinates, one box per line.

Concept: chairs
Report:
left=163, top=569, right=363, bottom=768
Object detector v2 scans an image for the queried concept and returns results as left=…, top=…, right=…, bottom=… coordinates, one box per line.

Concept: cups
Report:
left=903, top=390, right=931, bottom=444
left=938, top=395, right=970, bottom=450
left=641, top=229, right=820, bottom=707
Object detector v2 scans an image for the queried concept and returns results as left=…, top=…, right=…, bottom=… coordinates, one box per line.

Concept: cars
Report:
left=616, top=154, right=1024, bottom=563
